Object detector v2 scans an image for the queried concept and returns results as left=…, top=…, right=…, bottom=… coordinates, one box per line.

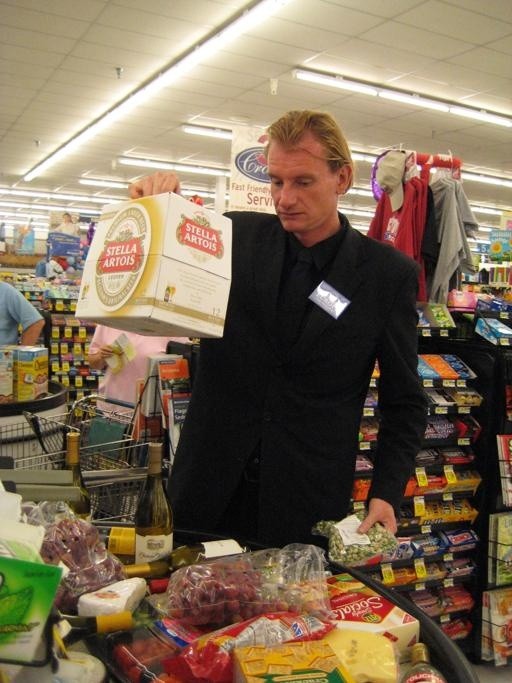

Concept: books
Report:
left=132, top=353, right=190, bottom=476
left=479, top=435, right=512, bottom=666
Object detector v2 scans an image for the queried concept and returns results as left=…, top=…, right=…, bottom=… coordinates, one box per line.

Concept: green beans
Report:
left=315, top=508, right=398, bottom=564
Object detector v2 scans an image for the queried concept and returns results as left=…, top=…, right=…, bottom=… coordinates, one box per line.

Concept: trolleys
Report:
left=0, top=395, right=171, bottom=525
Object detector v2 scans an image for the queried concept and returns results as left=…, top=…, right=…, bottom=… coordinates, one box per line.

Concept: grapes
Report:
left=163, top=558, right=316, bottom=626
left=40, top=516, right=124, bottom=614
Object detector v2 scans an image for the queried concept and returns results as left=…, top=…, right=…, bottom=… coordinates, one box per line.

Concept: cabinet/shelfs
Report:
left=338, top=337, right=496, bottom=666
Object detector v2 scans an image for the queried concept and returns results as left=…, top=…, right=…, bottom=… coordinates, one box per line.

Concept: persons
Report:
left=126, top=110, right=428, bottom=561
left=0, top=278, right=46, bottom=345
left=87, top=325, right=192, bottom=520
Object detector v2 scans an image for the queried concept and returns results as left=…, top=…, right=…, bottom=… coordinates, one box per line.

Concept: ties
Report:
left=273, top=248, right=313, bottom=355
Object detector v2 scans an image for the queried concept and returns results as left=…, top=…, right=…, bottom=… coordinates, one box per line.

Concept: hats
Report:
left=374, top=150, right=406, bottom=212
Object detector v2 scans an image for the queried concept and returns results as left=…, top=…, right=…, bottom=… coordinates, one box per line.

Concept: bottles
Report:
left=87, top=525, right=246, bottom=578
left=51, top=610, right=150, bottom=649
left=62, top=431, right=93, bottom=522
left=136, top=441, right=173, bottom=560
